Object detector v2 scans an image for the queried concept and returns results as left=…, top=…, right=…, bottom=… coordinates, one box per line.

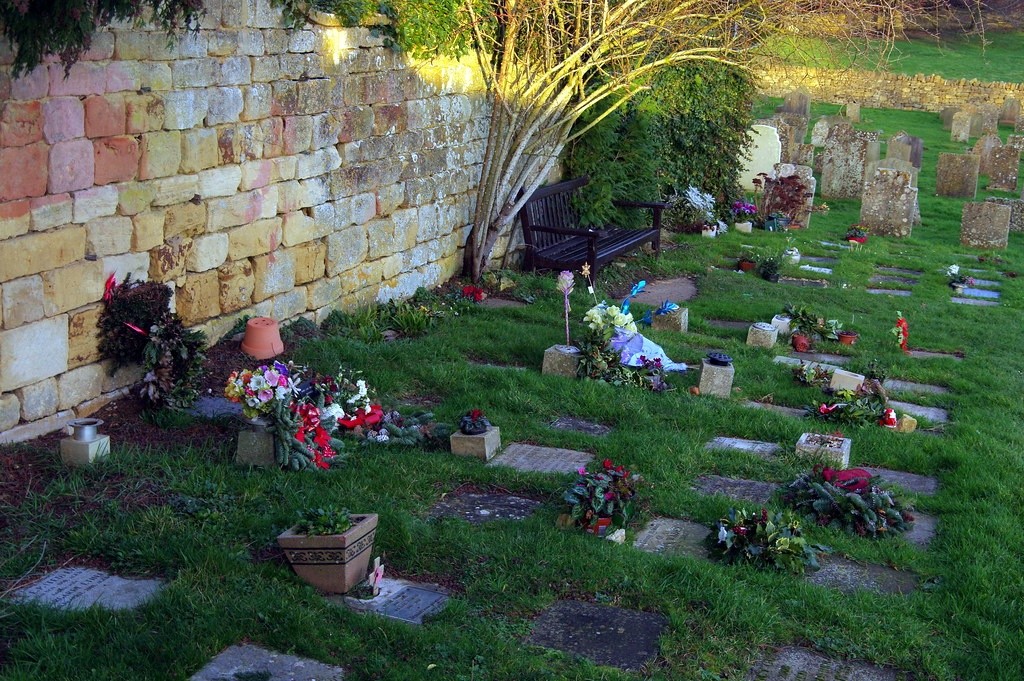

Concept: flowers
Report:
left=782, top=459, right=914, bottom=542
left=226, top=201, right=981, bottom=489
left=702, top=504, right=829, bottom=579
left=562, top=458, right=645, bottom=535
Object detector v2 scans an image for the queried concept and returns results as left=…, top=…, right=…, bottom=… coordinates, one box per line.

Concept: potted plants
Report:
left=796, top=433, right=851, bottom=471
left=701, top=220, right=716, bottom=237
left=790, top=329, right=809, bottom=352
left=838, top=331, right=858, bottom=344
left=776, top=213, right=790, bottom=227
left=764, top=258, right=779, bottom=283
left=278, top=506, right=379, bottom=594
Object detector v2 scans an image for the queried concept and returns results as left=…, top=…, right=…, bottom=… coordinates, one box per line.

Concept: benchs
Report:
left=514, top=171, right=668, bottom=291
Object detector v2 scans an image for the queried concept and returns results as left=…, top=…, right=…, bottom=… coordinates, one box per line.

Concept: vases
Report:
left=734, top=221, right=752, bottom=234
left=738, top=259, right=756, bottom=272
left=952, top=282, right=968, bottom=295
left=448, top=430, right=500, bottom=461
left=583, top=509, right=613, bottom=536
left=848, top=235, right=866, bottom=244
left=829, top=369, right=865, bottom=392
left=237, top=317, right=285, bottom=361
left=240, top=415, right=273, bottom=434
